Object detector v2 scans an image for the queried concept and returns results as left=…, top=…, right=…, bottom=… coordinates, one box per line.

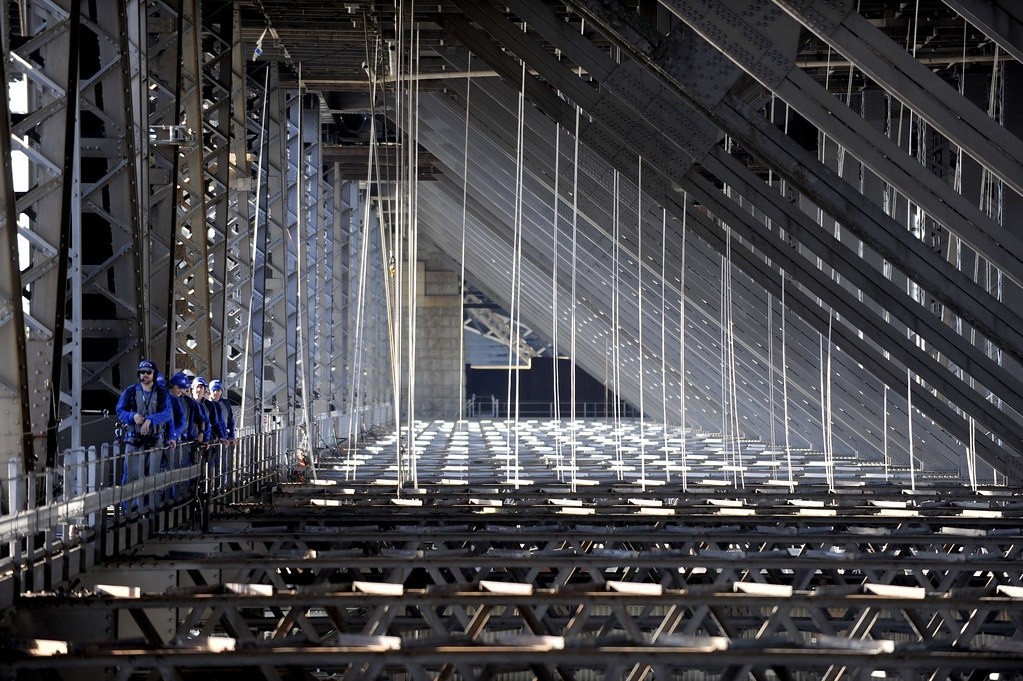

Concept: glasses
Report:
left=139, top=370, right=152, bottom=375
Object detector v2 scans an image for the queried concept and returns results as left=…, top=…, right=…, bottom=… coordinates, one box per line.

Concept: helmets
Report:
left=193, top=377, right=208, bottom=386
left=136, top=359, right=156, bottom=373
left=209, top=379, right=222, bottom=390
left=169, top=371, right=192, bottom=388
left=156, top=371, right=166, bottom=386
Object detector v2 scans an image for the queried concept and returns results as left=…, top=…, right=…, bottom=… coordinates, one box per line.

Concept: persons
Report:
left=116, top=364, right=172, bottom=512
left=157, top=372, right=235, bottom=503
